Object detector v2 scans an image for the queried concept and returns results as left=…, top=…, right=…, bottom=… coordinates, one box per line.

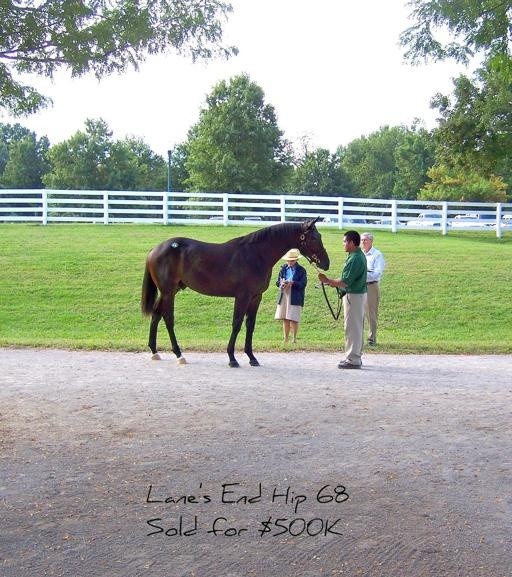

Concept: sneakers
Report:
left=340, top=359, right=362, bottom=365
left=337, top=362, right=361, bottom=369
left=368, top=342, right=376, bottom=346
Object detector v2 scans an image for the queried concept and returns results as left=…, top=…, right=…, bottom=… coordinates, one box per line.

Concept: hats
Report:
left=281, top=248, right=303, bottom=261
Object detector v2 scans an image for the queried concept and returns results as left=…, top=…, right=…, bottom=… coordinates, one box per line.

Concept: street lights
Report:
left=166, top=148, right=176, bottom=217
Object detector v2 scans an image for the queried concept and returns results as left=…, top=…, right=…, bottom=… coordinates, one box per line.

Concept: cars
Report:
left=209, top=214, right=512, bottom=228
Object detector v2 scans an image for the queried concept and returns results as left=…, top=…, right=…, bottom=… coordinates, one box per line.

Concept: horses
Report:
left=139, top=215, right=330, bottom=369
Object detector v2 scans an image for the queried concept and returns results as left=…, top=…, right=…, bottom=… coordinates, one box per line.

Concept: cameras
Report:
left=280, top=280, right=289, bottom=289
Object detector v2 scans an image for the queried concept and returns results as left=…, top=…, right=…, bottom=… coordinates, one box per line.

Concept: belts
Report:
left=367, top=281, right=378, bottom=284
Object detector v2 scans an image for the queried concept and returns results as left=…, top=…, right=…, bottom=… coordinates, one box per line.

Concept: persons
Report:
left=359, top=232, right=385, bottom=343
left=276, top=247, right=308, bottom=346
left=317, top=229, right=367, bottom=369
